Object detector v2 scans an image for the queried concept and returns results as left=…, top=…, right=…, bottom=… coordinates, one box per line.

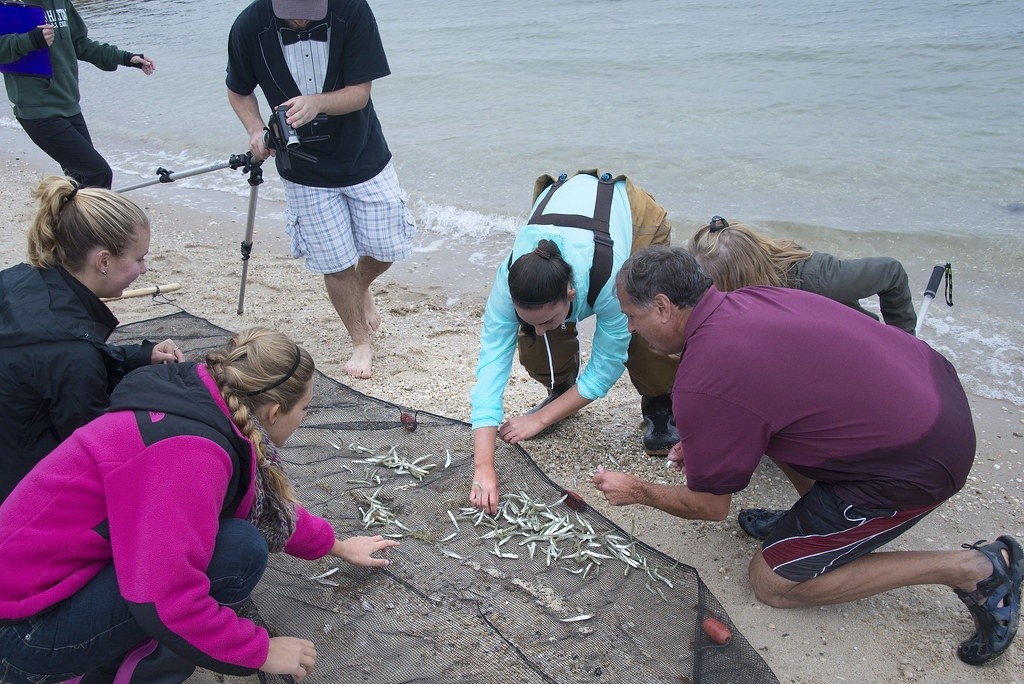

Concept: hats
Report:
left=272, top=0, right=328, bottom=21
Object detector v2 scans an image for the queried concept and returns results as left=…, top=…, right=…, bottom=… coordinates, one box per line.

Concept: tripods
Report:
left=112, top=155, right=263, bottom=316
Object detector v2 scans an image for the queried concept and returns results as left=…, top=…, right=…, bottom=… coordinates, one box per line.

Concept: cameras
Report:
left=268, top=104, right=301, bottom=152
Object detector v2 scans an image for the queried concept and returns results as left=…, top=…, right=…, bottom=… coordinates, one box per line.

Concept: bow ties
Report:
left=280, top=24, right=327, bottom=45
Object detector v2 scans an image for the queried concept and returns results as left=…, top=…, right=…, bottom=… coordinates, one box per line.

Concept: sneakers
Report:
left=738, top=508, right=787, bottom=540
left=524, top=388, right=568, bottom=433
left=641, top=399, right=681, bottom=455
left=953, top=535, right=1023, bottom=664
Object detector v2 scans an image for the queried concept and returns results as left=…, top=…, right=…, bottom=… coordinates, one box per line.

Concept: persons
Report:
left=1, top=0, right=174, bottom=190
left=224, top=0, right=416, bottom=379
left=469, top=168, right=1024, bottom=665
left=0, top=187, right=400, bottom=684
